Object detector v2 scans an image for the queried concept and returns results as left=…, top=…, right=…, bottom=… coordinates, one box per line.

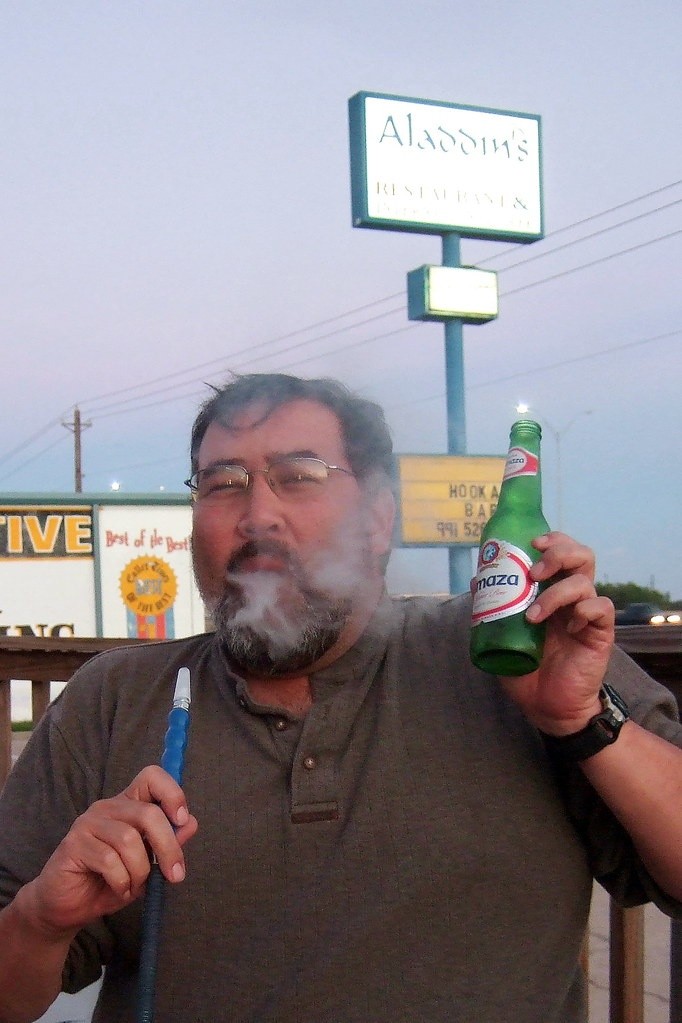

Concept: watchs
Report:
left=536, top=680, right=631, bottom=766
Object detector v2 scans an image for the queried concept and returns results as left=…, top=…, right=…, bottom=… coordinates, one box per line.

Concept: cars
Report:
left=614, top=601, right=681, bottom=626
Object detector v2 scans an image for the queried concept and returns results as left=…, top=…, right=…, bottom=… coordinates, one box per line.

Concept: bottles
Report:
left=468, top=420, right=550, bottom=676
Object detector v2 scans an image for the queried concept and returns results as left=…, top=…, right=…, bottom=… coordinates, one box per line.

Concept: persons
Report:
left=0, top=371, right=681, bottom=1023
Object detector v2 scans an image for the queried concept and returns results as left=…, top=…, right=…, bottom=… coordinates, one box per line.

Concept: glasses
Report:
left=183, top=458, right=359, bottom=507
